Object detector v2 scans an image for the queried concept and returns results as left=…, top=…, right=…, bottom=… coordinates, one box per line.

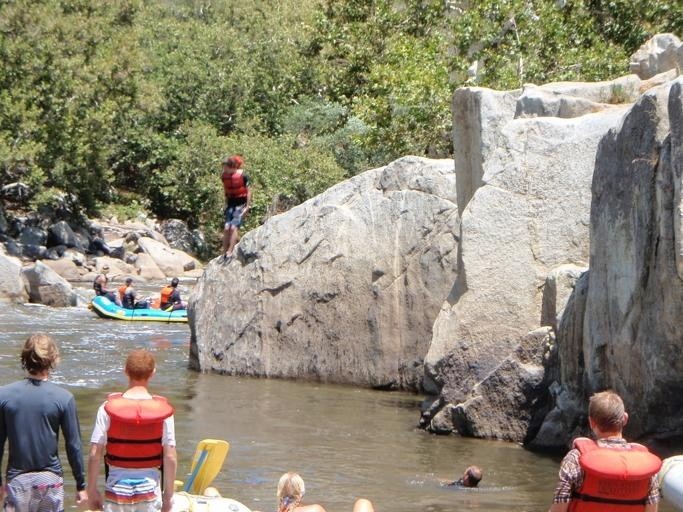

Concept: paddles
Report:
left=188, top=439, right=230, bottom=496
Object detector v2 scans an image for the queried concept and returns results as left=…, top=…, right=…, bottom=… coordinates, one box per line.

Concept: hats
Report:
left=172, top=278, right=179, bottom=286
left=100, top=265, right=110, bottom=274
left=221, top=156, right=243, bottom=169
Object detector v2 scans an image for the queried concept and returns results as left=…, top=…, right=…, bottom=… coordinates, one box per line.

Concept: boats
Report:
left=88, top=294, right=189, bottom=325
left=164, top=482, right=250, bottom=512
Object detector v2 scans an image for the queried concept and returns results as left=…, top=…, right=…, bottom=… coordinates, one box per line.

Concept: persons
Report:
left=219, top=154, right=251, bottom=266
left=159, top=276, right=188, bottom=312
left=93, top=264, right=121, bottom=307
left=85, top=348, right=178, bottom=511
left=446, top=465, right=484, bottom=488
left=546, top=389, right=664, bottom=512
left=0, top=331, right=89, bottom=511
left=119, top=277, right=145, bottom=310
left=274, top=470, right=375, bottom=512
